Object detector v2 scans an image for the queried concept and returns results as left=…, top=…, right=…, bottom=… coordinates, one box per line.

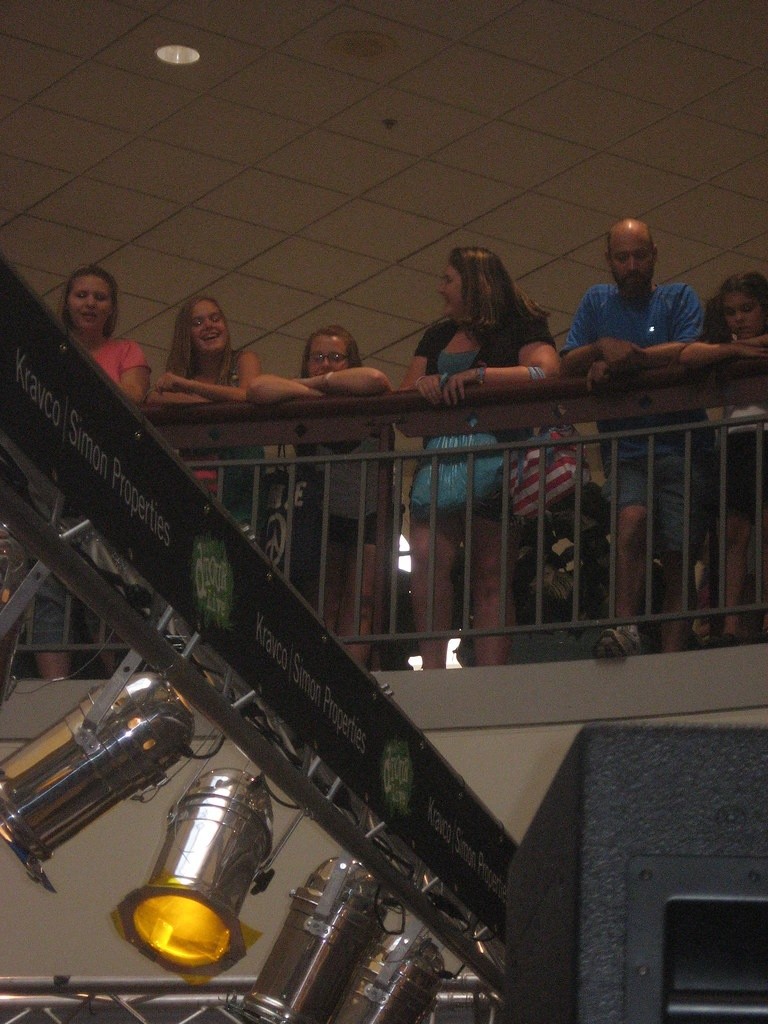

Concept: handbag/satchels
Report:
left=253, top=443, right=328, bottom=599
left=509, top=422, right=593, bottom=517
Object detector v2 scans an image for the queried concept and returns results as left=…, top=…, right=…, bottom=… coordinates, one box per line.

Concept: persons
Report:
left=30, top=217, right=768, bottom=681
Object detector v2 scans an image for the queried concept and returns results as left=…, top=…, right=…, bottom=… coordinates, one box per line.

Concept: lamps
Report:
left=0, top=671, right=495, bottom=1024
left=153, top=24, right=201, bottom=66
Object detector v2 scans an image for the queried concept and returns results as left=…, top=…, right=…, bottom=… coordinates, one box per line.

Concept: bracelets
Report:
left=415, top=375, right=426, bottom=387
left=322, top=371, right=333, bottom=390
left=477, top=361, right=486, bottom=385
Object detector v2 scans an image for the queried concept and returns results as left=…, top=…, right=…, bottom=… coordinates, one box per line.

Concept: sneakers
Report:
left=593, top=625, right=642, bottom=656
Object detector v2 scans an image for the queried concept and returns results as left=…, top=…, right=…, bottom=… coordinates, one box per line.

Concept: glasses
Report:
left=305, top=352, right=347, bottom=363
